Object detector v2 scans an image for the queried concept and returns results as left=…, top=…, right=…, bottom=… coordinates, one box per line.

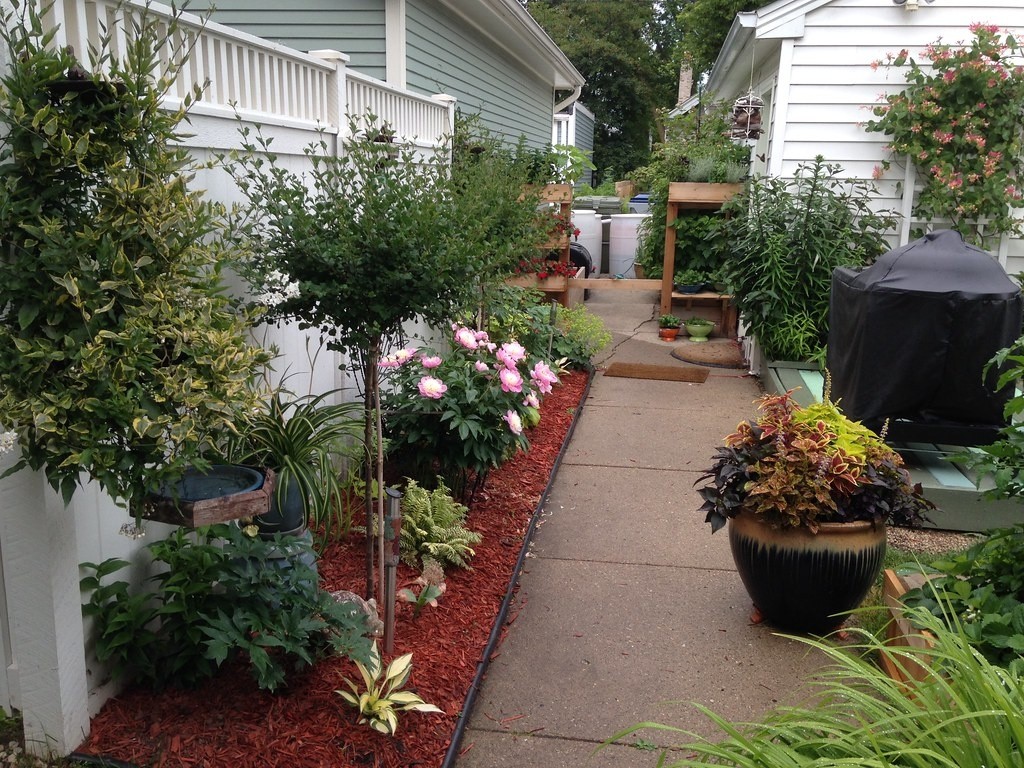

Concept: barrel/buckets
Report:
left=570, top=209, right=598, bottom=277
left=595, top=214, right=603, bottom=274
left=608, top=214, right=654, bottom=277
left=570, top=242, right=592, bottom=279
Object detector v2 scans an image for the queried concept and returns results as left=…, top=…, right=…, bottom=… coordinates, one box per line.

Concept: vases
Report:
left=254, top=531, right=317, bottom=621
left=534, top=229, right=569, bottom=246
left=505, top=271, right=567, bottom=292
left=729, top=510, right=889, bottom=638
left=130, top=460, right=274, bottom=530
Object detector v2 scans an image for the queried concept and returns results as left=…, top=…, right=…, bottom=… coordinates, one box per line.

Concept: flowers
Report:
left=855, top=22, right=1024, bottom=251
left=544, top=212, right=580, bottom=237
left=515, top=260, right=576, bottom=279
left=372, top=318, right=571, bottom=505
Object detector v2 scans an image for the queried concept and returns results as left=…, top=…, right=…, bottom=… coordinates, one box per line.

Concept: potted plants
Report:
left=673, top=269, right=706, bottom=295
left=707, top=270, right=734, bottom=291
left=634, top=237, right=650, bottom=278
left=874, top=521, right=1024, bottom=748
left=239, top=388, right=367, bottom=539
left=657, top=314, right=682, bottom=342
left=684, top=316, right=714, bottom=342
